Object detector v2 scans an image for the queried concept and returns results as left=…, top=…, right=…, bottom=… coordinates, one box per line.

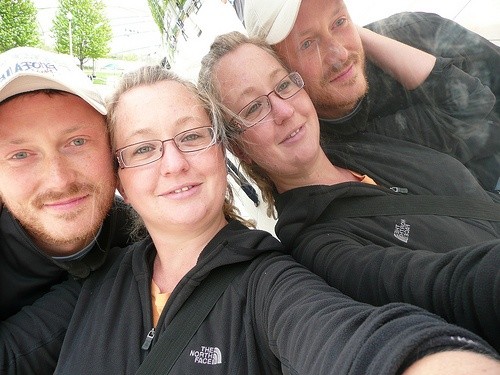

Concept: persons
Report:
left=0, top=45, right=147, bottom=322
left=197, top=28, right=499, bottom=352
left=244, top=0, right=499, bottom=196
left=0, top=67, right=499, bottom=375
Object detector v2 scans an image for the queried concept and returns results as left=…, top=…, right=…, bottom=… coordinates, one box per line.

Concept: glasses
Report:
left=114, top=125, right=218, bottom=169
left=226, top=71, right=305, bottom=137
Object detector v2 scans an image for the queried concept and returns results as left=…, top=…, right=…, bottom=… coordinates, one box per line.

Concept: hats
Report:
left=242, top=0, right=304, bottom=46
left=0, top=46, right=108, bottom=115
